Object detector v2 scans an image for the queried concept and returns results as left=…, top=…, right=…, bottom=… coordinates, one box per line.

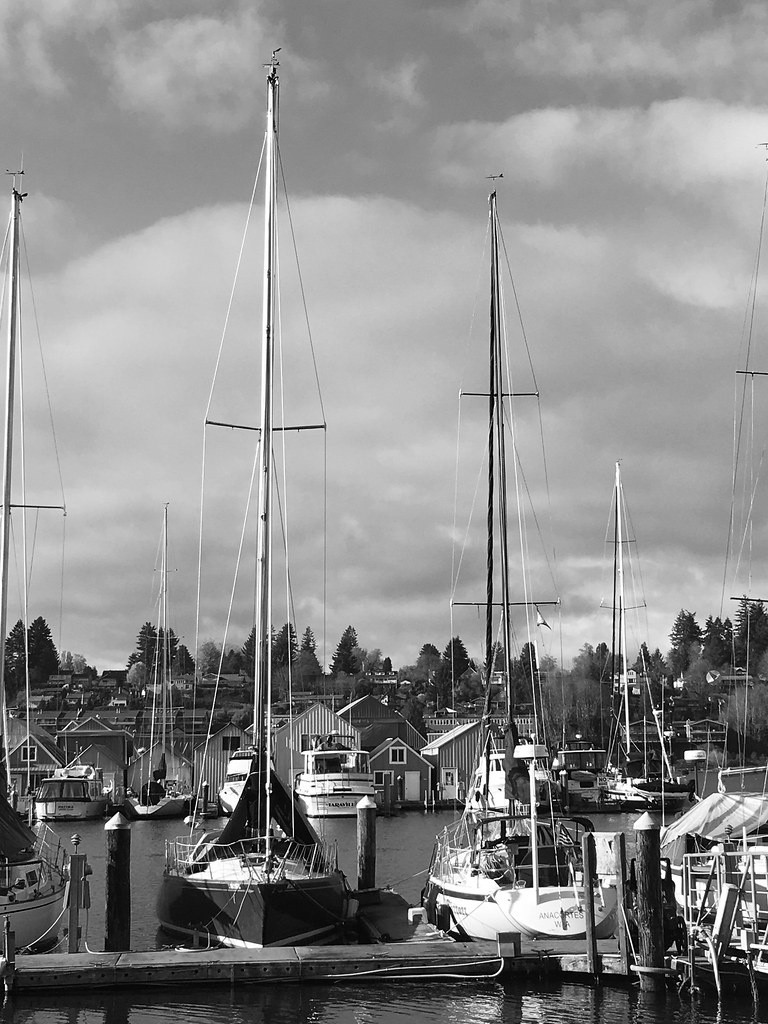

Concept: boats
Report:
left=34, top=730, right=115, bottom=822
left=293, top=731, right=376, bottom=818
left=469, top=732, right=563, bottom=819
left=219, top=743, right=276, bottom=816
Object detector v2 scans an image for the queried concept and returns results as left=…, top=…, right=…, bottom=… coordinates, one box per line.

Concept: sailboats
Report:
left=660, top=139, right=768, bottom=930
left=605, top=462, right=695, bottom=814
left=422, top=175, right=620, bottom=941
left=120, top=502, right=196, bottom=820
left=1, top=161, right=68, bottom=956
left=158, top=47, right=352, bottom=952
